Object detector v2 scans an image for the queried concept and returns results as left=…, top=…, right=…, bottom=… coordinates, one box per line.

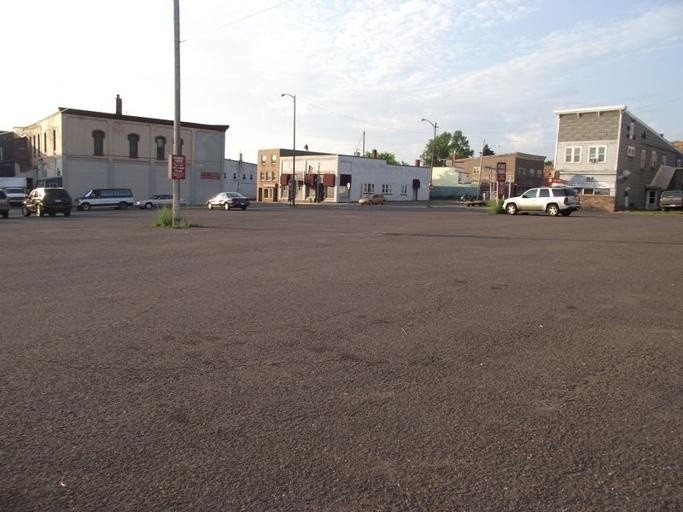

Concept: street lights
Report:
left=420, top=119, right=438, bottom=200
left=280, top=94, right=297, bottom=207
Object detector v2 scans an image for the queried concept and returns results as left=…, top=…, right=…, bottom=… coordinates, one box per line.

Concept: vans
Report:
left=73, top=188, right=133, bottom=211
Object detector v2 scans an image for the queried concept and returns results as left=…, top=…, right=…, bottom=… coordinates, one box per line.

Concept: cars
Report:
left=658, top=190, right=683, bottom=210
left=205, top=192, right=248, bottom=211
left=134, top=195, right=186, bottom=209
left=358, top=193, right=386, bottom=205
left=20, top=187, right=72, bottom=216
left=0, top=189, right=9, bottom=218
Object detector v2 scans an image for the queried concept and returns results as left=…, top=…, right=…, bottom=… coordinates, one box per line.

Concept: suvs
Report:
left=501, top=185, right=581, bottom=216
